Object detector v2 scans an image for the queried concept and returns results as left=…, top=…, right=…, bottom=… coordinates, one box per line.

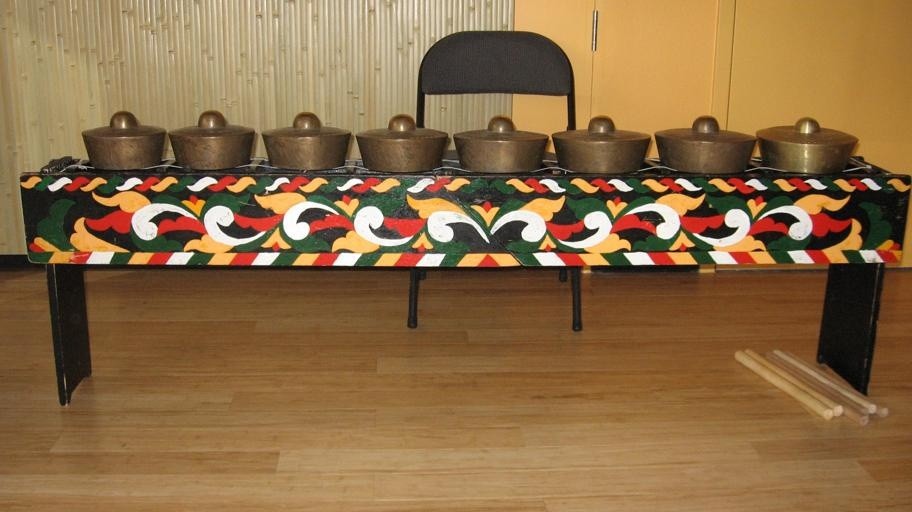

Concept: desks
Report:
left=19, top=158, right=912, bottom=409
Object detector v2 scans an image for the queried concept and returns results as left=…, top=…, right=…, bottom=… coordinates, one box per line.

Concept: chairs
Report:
left=407, top=30, right=582, bottom=332
